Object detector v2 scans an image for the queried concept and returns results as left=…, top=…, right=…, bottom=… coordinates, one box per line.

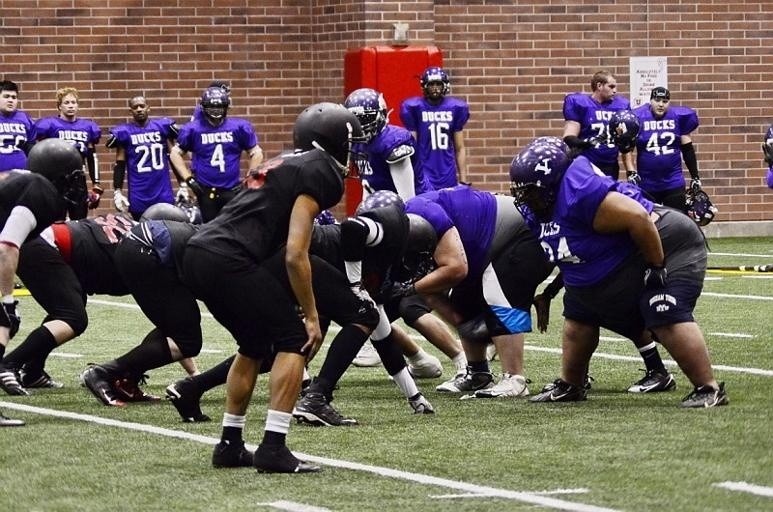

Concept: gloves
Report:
left=642, top=260, right=669, bottom=290
left=0, top=301, right=20, bottom=339
left=231, top=184, right=243, bottom=194
left=185, top=176, right=205, bottom=198
left=113, top=188, right=130, bottom=212
left=176, top=188, right=190, bottom=207
left=408, top=392, right=435, bottom=414
left=390, top=278, right=416, bottom=302
left=86, top=184, right=103, bottom=210
left=626, top=171, right=641, bottom=184
left=690, top=176, right=701, bottom=196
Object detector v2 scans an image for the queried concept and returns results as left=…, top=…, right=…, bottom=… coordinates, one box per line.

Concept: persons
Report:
left=0, top=79, right=434, bottom=472
left=761, top=125, right=772, bottom=191
left=341, top=70, right=728, bottom=413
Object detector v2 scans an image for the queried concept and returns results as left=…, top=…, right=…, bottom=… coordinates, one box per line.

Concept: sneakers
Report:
left=353, top=343, right=383, bottom=367
left=164, top=377, right=210, bottom=422
left=475, top=371, right=530, bottom=398
left=388, top=355, right=442, bottom=381
left=0, top=411, right=25, bottom=427
left=18, top=371, right=65, bottom=388
left=0, top=358, right=31, bottom=396
left=292, top=393, right=358, bottom=426
left=542, top=375, right=594, bottom=392
left=213, top=441, right=255, bottom=468
left=681, top=382, right=729, bottom=408
left=436, top=366, right=495, bottom=393
left=83, top=363, right=127, bottom=407
left=627, top=369, right=676, bottom=394
left=531, top=375, right=595, bottom=402
left=111, top=374, right=161, bottom=402
left=485, top=345, right=497, bottom=362
left=255, top=445, right=320, bottom=473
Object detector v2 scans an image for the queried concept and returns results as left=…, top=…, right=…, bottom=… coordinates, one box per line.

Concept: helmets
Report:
left=762, top=124, right=773, bottom=168
left=510, top=142, right=570, bottom=227
left=29, top=138, right=88, bottom=221
left=139, top=203, right=190, bottom=222
left=202, top=87, right=229, bottom=126
left=356, top=190, right=405, bottom=216
left=393, top=213, right=437, bottom=287
left=293, top=102, right=370, bottom=179
left=182, top=205, right=203, bottom=225
left=345, top=89, right=387, bottom=142
left=609, top=111, right=640, bottom=153
left=421, top=68, right=449, bottom=100
left=529, top=137, right=572, bottom=160
left=679, top=188, right=718, bottom=226
